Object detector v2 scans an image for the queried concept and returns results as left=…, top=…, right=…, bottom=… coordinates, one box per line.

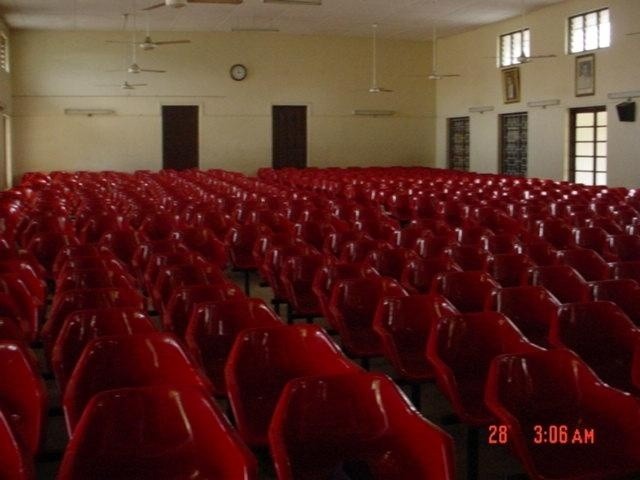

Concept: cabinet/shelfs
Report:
left=501, top=113, right=528, bottom=177
left=447, top=116, right=469, bottom=172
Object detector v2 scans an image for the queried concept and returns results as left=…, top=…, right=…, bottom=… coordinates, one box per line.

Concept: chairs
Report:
left=0, top=165, right=640, bottom=479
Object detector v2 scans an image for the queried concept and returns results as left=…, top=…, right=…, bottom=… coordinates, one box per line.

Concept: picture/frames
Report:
left=574, top=53, right=595, bottom=97
left=501, top=67, right=521, bottom=104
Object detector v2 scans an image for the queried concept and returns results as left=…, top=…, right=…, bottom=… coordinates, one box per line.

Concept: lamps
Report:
left=355, top=110, right=393, bottom=116
left=66, top=109, right=113, bottom=115
left=468, top=106, right=494, bottom=113
left=608, top=91, right=640, bottom=98
left=528, top=100, right=559, bottom=106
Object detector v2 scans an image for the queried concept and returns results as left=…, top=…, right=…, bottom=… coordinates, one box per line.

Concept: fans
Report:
left=498, top=0, right=555, bottom=62
left=359, top=23, right=393, bottom=93
left=105, top=10, right=190, bottom=50
left=109, top=11, right=167, bottom=73
left=102, top=12, right=148, bottom=89
left=404, top=18, right=460, bottom=79
left=144, top=0, right=243, bottom=15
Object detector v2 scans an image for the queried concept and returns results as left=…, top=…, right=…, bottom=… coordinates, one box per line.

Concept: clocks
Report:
left=230, top=64, right=247, bottom=81
